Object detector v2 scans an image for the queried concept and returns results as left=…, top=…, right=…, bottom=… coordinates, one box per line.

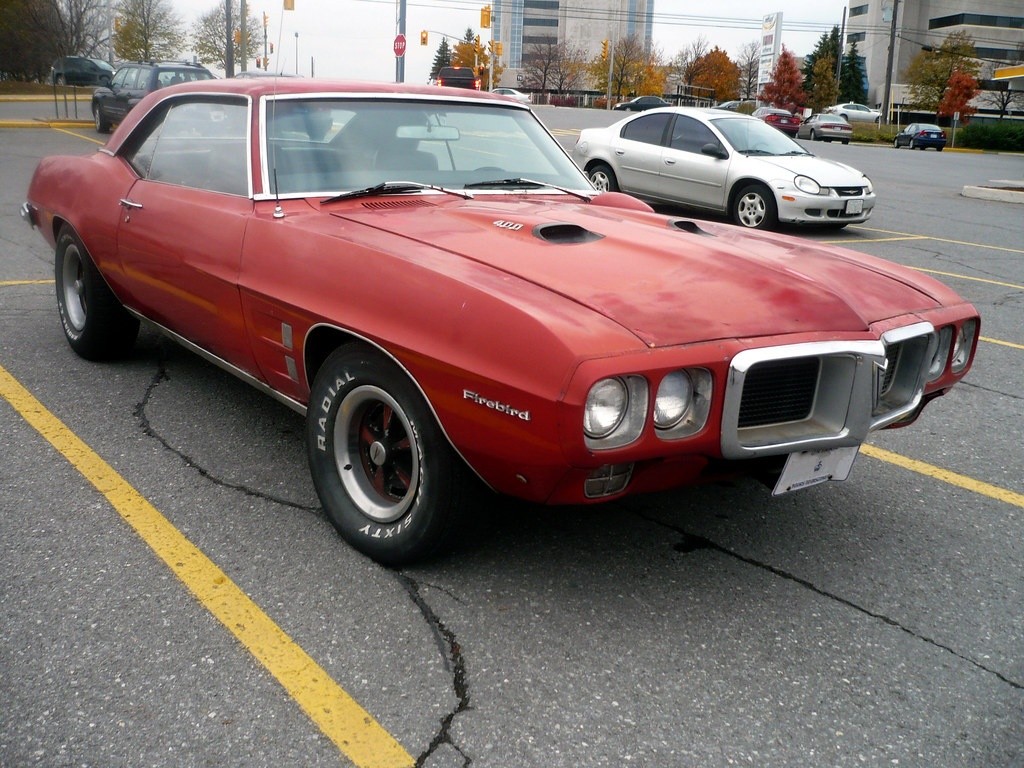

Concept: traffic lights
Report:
left=488, top=39, right=494, bottom=53
left=472, top=66, right=478, bottom=77
left=247, top=11, right=273, bottom=68
left=600, top=39, right=609, bottom=60
left=420, top=31, right=428, bottom=46
left=479, top=66, right=484, bottom=76
left=472, top=35, right=480, bottom=54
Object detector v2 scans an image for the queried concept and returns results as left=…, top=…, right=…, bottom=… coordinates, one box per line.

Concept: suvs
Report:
left=91, top=58, right=219, bottom=133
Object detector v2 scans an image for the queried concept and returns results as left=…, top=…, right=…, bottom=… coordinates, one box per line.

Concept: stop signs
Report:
left=394, top=34, right=407, bottom=56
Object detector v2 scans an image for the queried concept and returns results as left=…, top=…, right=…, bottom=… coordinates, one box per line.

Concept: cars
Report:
left=435, top=66, right=480, bottom=90
left=895, top=121, right=946, bottom=152
left=488, top=88, right=533, bottom=104
left=18, top=75, right=983, bottom=568
left=710, top=99, right=748, bottom=111
left=568, top=105, right=877, bottom=233
left=49, top=54, right=116, bottom=88
left=749, top=106, right=801, bottom=137
left=824, top=103, right=884, bottom=124
left=612, top=95, right=672, bottom=112
left=233, top=70, right=333, bottom=141
left=797, top=112, right=856, bottom=145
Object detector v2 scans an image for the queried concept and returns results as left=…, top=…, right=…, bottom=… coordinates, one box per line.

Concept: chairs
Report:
left=373, top=138, right=437, bottom=171
left=271, top=145, right=345, bottom=175
left=170, top=76, right=182, bottom=85
